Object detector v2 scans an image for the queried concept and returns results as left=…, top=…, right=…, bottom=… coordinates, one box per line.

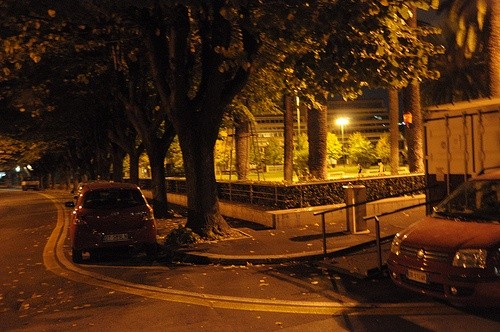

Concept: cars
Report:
left=388, top=166, right=500, bottom=316
left=70, top=182, right=83, bottom=206
left=21, top=176, right=40, bottom=191
left=65, top=183, right=157, bottom=264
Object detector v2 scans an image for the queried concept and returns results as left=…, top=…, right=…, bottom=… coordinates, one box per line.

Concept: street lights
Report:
left=336, top=118, right=349, bottom=168
